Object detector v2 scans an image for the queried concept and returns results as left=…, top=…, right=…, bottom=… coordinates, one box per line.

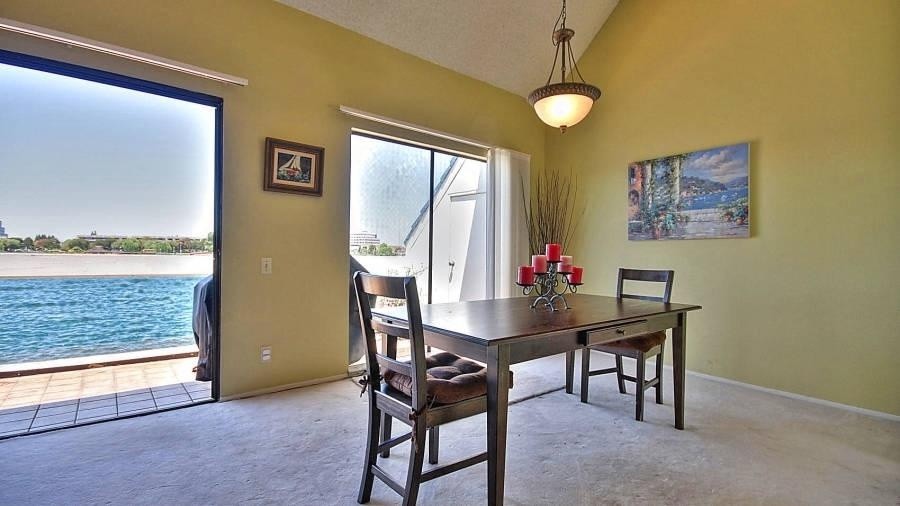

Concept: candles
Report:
left=517, top=243, right=583, bottom=284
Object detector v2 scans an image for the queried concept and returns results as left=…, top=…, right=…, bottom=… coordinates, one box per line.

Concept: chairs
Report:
left=581, top=267, right=674, bottom=422
left=352, top=270, right=514, bottom=506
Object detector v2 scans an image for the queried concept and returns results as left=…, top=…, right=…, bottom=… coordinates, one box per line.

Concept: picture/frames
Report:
left=263, top=137, right=325, bottom=197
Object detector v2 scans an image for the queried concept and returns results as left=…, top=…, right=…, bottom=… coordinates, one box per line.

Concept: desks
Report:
left=370, top=291, right=703, bottom=506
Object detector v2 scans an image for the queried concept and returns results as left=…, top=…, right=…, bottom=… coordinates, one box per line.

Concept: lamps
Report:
left=527, top=0, right=601, bottom=137
